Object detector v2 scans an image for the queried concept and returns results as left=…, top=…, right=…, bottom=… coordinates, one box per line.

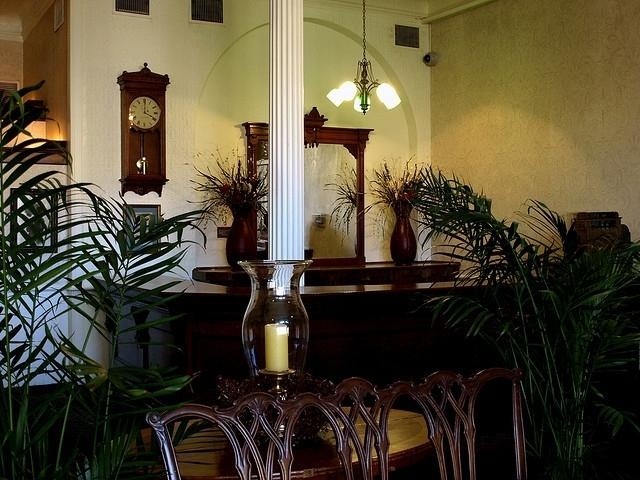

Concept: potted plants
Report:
left=360, top=155, right=445, bottom=265
left=185, top=143, right=271, bottom=268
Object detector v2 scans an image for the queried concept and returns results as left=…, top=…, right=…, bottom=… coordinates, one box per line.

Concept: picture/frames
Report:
left=11, top=188, right=58, bottom=254
left=123, top=205, right=162, bottom=255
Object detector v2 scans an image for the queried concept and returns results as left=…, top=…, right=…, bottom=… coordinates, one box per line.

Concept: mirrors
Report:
left=242, top=122, right=374, bottom=266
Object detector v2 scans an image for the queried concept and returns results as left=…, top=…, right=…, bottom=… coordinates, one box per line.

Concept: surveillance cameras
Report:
left=423, top=52, right=437, bottom=66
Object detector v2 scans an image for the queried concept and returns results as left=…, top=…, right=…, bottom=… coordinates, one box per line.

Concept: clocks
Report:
left=116, top=61, right=169, bottom=197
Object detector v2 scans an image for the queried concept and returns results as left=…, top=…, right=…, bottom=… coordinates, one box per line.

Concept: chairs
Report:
left=144, top=367, right=532, bottom=479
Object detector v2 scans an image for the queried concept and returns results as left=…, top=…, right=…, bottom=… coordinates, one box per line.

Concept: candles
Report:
left=265, top=323, right=290, bottom=372
